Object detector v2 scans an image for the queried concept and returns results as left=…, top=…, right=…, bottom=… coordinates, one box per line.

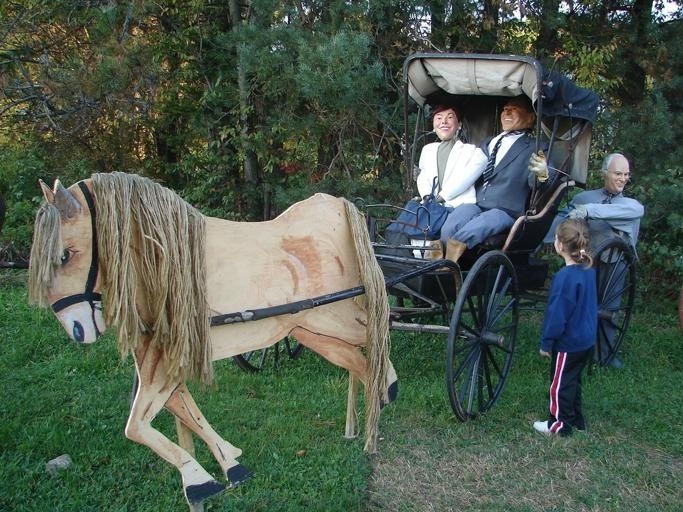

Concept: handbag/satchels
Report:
left=386, top=176, right=449, bottom=240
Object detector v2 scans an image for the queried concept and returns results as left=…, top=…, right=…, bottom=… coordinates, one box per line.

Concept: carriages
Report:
left=24, top=53, right=637, bottom=505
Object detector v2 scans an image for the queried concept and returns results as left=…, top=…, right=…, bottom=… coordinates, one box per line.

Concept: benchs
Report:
left=462, top=181, right=586, bottom=267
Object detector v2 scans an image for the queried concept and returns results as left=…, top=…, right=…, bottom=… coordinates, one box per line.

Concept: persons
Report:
left=540, top=153, right=646, bottom=371
left=424, top=97, right=556, bottom=271
left=531, top=219, right=600, bottom=439
left=407, top=102, right=490, bottom=261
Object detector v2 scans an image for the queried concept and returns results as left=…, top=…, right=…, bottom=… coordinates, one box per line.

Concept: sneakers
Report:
left=533, top=421, right=548, bottom=433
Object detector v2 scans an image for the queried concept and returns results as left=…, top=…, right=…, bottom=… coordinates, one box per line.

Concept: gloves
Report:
left=527, top=149, right=549, bottom=182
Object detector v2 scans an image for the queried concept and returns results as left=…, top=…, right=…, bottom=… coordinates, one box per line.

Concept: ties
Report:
left=482, top=130, right=522, bottom=190
left=602, top=192, right=638, bottom=263
